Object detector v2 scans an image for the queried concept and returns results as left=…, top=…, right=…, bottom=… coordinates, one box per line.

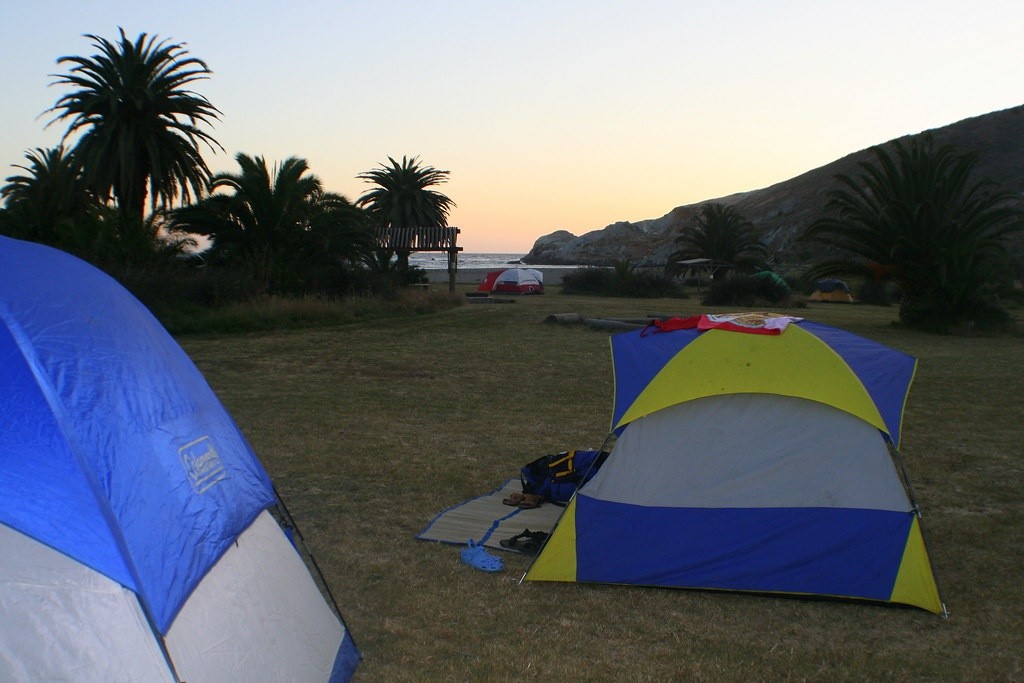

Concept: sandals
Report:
left=460, top=539, right=504, bottom=571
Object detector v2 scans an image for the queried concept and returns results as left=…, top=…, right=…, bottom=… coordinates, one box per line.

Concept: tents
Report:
left=0, top=236, right=367, bottom=683
left=518, top=311, right=949, bottom=616
left=477, top=266, right=545, bottom=296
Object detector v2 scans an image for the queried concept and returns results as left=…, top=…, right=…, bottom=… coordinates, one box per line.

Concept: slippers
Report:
left=519, top=531, right=549, bottom=552
left=503, top=492, right=526, bottom=506
left=518, top=494, right=543, bottom=508
left=500, top=529, right=533, bottom=548
